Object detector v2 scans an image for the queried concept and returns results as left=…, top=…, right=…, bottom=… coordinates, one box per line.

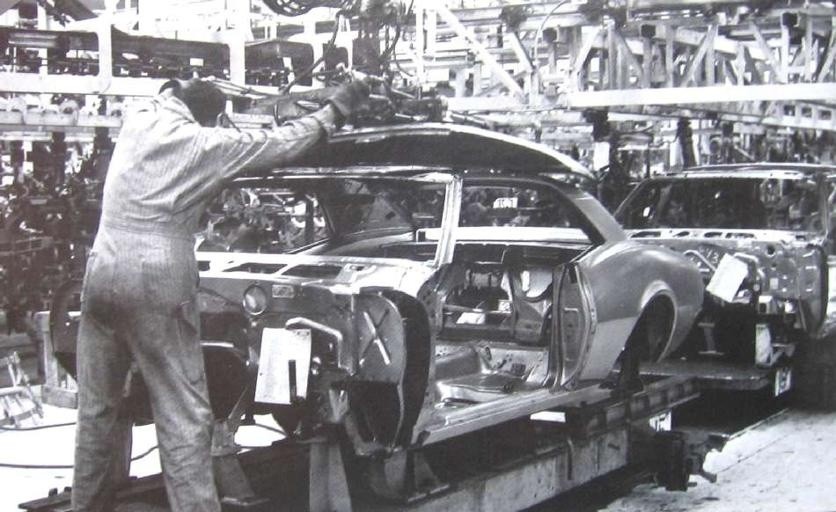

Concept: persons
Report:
left=69, top=78, right=372, bottom=512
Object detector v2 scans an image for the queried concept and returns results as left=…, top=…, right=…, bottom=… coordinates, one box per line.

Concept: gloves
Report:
left=321, top=80, right=370, bottom=126
